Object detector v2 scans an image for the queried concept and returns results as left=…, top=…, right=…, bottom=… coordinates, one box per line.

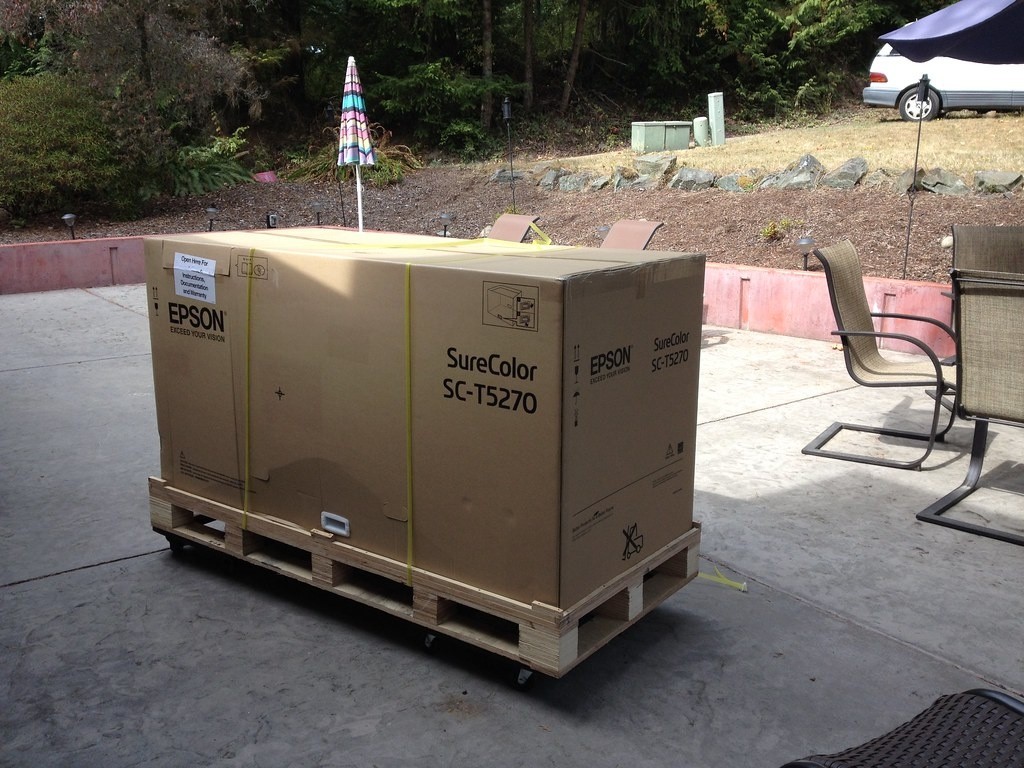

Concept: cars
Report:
left=863, top=20, right=1023, bottom=121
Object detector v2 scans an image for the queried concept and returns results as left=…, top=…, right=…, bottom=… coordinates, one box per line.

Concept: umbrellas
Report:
left=337, top=56, right=378, bottom=232
left=878, top=0, right=1024, bottom=64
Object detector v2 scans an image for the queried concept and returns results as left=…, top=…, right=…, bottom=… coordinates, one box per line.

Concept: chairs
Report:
left=801, top=239, right=961, bottom=470
left=486, top=213, right=541, bottom=244
left=599, top=219, right=663, bottom=252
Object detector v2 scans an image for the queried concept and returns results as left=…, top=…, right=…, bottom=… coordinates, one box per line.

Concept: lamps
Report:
left=798, top=237, right=814, bottom=254
left=440, top=212, right=450, bottom=226
left=62, top=214, right=76, bottom=227
left=206, top=204, right=219, bottom=220
left=313, top=201, right=323, bottom=213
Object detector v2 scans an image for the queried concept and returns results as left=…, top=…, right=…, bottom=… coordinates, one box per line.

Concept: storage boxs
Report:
left=917, top=269, right=1024, bottom=547
left=924, top=224, right=1024, bottom=416
left=145, top=226, right=708, bottom=611
